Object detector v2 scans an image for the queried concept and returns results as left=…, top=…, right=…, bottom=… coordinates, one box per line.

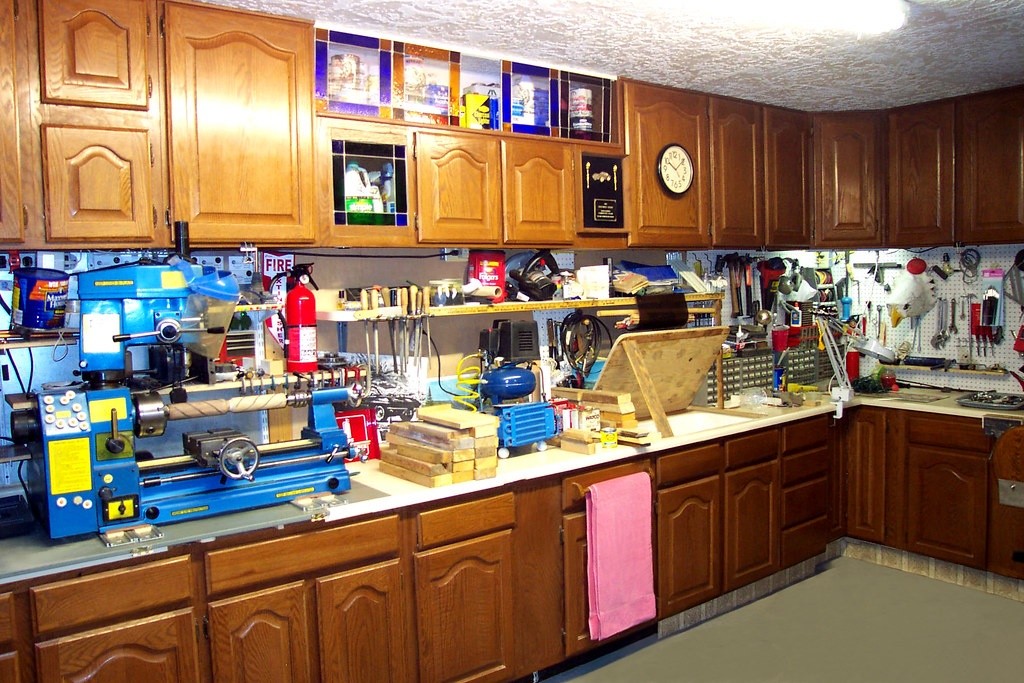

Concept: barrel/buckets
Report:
left=10, top=268, right=69, bottom=333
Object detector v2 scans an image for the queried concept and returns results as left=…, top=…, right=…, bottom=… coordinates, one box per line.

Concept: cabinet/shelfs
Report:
left=0, top=0, right=1024, bottom=683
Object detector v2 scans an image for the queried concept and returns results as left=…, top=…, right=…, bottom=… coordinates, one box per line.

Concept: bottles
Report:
left=344, top=160, right=394, bottom=213
left=337, top=289, right=345, bottom=312
left=847, top=349, right=860, bottom=384
left=882, top=369, right=896, bottom=391
left=790, top=302, right=802, bottom=327
left=736, top=326, right=745, bottom=357
left=943, top=253, right=954, bottom=277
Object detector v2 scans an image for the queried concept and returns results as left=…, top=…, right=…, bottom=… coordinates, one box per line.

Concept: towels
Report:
left=586, top=471, right=657, bottom=642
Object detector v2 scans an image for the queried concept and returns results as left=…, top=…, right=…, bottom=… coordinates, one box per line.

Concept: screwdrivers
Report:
left=361, top=285, right=432, bottom=373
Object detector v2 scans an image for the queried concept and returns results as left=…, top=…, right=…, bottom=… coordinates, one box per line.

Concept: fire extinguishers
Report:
left=270, top=261, right=318, bottom=373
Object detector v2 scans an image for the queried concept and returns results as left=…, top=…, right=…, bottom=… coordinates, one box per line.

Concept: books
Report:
left=467, top=250, right=506, bottom=303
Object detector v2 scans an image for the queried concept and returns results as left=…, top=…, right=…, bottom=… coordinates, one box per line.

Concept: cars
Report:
left=334, top=385, right=422, bottom=422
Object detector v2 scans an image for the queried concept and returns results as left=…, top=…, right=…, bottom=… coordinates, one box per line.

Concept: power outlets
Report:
left=440, top=247, right=469, bottom=262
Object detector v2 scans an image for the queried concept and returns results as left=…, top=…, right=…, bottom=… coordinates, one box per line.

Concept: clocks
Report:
left=656, top=143, right=694, bottom=197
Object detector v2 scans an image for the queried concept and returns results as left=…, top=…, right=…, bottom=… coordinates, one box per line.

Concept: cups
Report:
left=722, top=344, right=731, bottom=358
left=772, top=326, right=790, bottom=351
left=741, top=387, right=767, bottom=413
